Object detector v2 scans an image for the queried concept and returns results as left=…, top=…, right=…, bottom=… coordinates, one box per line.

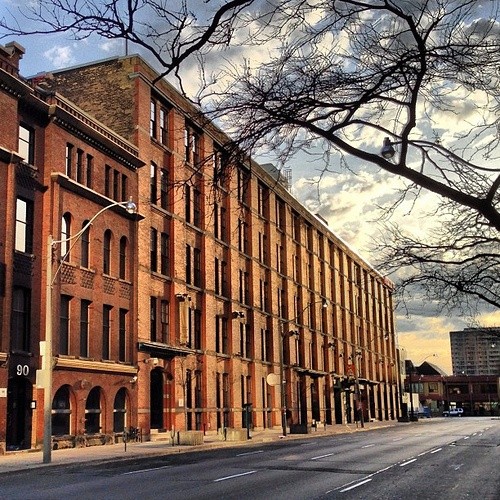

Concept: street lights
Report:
left=279, top=301, right=328, bottom=438
left=410, top=353, right=437, bottom=423
left=43, top=194, right=135, bottom=464
left=354, top=335, right=385, bottom=432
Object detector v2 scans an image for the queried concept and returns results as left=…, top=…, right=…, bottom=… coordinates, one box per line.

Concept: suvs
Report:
left=442, top=407, right=464, bottom=417
